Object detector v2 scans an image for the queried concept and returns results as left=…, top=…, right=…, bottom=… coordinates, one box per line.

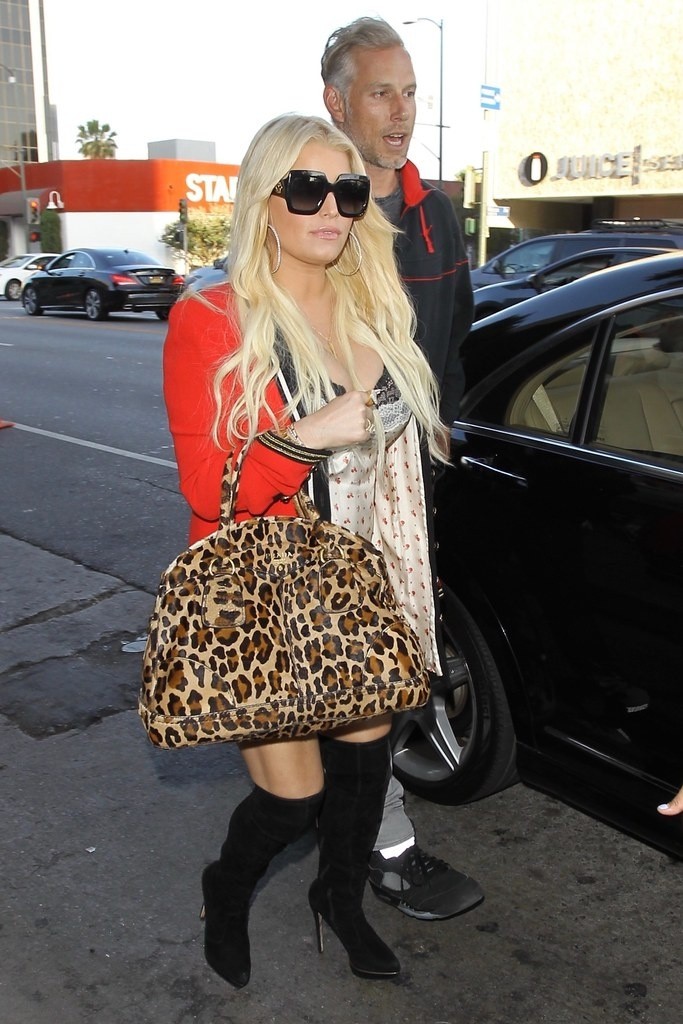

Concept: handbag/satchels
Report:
left=137, top=433, right=432, bottom=748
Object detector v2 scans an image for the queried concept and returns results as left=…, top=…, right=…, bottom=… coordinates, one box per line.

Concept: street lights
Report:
left=402, top=17, right=444, bottom=188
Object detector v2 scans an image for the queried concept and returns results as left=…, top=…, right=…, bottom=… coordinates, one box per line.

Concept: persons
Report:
left=162, top=116, right=429, bottom=989
left=321, top=18, right=502, bottom=920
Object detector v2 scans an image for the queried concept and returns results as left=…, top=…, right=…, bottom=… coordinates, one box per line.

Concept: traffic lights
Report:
left=176, top=232, right=183, bottom=241
left=25, top=197, right=40, bottom=224
left=465, top=218, right=476, bottom=236
left=179, top=199, right=188, bottom=222
left=29, top=230, right=42, bottom=243
left=459, top=167, right=475, bottom=208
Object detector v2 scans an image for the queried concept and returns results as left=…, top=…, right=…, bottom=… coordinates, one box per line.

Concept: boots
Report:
left=201, top=773, right=326, bottom=988
left=308, top=731, right=401, bottom=979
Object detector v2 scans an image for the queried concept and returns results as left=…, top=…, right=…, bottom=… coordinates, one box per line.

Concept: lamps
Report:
left=46, top=189, right=65, bottom=212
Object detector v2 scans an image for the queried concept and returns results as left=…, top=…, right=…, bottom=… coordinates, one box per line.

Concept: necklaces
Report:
left=279, top=279, right=342, bottom=360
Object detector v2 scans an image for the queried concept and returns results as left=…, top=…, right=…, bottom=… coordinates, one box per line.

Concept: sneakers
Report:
left=366, top=843, right=485, bottom=921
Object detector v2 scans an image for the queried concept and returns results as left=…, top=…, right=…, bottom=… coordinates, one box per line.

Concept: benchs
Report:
left=522, top=351, right=683, bottom=454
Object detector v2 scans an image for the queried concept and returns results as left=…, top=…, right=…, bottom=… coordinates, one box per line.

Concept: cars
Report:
left=0, top=253, right=80, bottom=300
left=388, top=218, right=683, bottom=859
left=19, top=247, right=229, bottom=322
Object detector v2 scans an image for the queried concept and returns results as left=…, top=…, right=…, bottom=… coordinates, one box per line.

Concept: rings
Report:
left=366, top=419, right=376, bottom=434
left=366, top=397, right=374, bottom=407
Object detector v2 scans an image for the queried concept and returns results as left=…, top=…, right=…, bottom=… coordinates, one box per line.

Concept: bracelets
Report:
left=286, top=421, right=304, bottom=447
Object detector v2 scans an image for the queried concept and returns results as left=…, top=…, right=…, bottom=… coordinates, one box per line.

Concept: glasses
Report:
left=271, top=170, right=370, bottom=217
